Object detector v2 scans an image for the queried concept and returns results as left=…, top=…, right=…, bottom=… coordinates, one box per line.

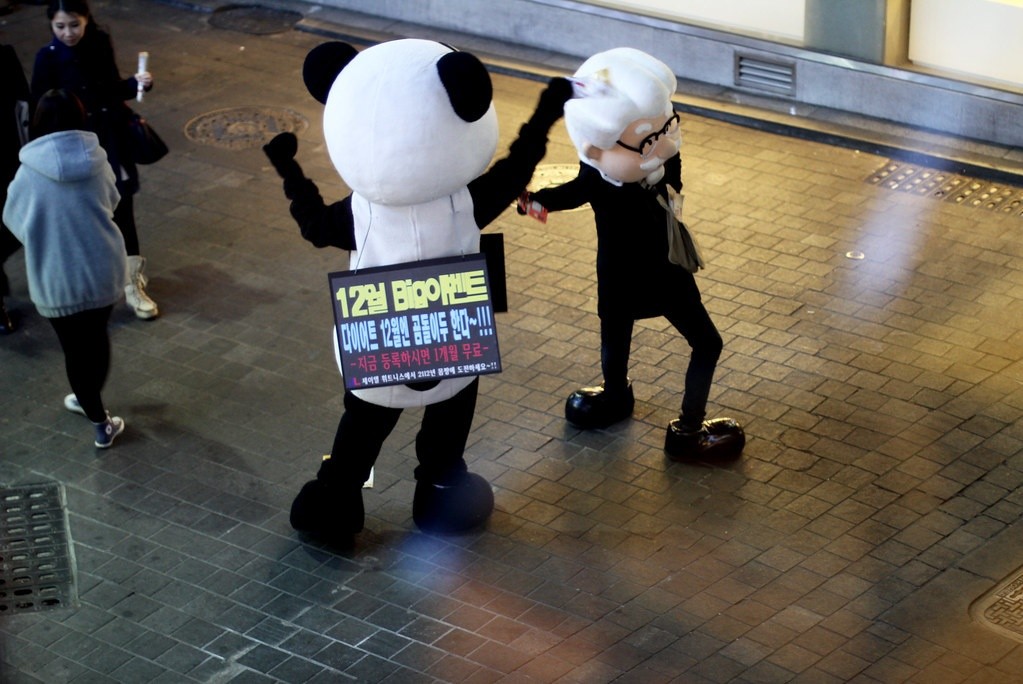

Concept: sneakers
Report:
left=63, top=393, right=87, bottom=418
left=91, top=410, right=123, bottom=448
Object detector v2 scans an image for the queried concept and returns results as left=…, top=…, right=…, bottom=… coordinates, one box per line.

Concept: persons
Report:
left=31, top=0, right=158, bottom=319
left=2, top=85, right=124, bottom=446
left=0, top=43, right=29, bottom=333
left=517, top=47, right=746, bottom=460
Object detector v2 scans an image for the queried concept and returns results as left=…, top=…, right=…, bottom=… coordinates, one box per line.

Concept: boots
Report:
left=121, top=254, right=157, bottom=318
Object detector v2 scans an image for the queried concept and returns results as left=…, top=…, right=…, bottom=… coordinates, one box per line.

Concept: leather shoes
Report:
left=0, top=307, right=13, bottom=333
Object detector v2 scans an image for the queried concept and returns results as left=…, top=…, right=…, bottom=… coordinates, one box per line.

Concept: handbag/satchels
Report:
left=122, top=104, right=170, bottom=168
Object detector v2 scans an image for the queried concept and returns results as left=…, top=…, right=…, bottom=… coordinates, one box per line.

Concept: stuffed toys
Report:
left=262, top=38, right=574, bottom=531
